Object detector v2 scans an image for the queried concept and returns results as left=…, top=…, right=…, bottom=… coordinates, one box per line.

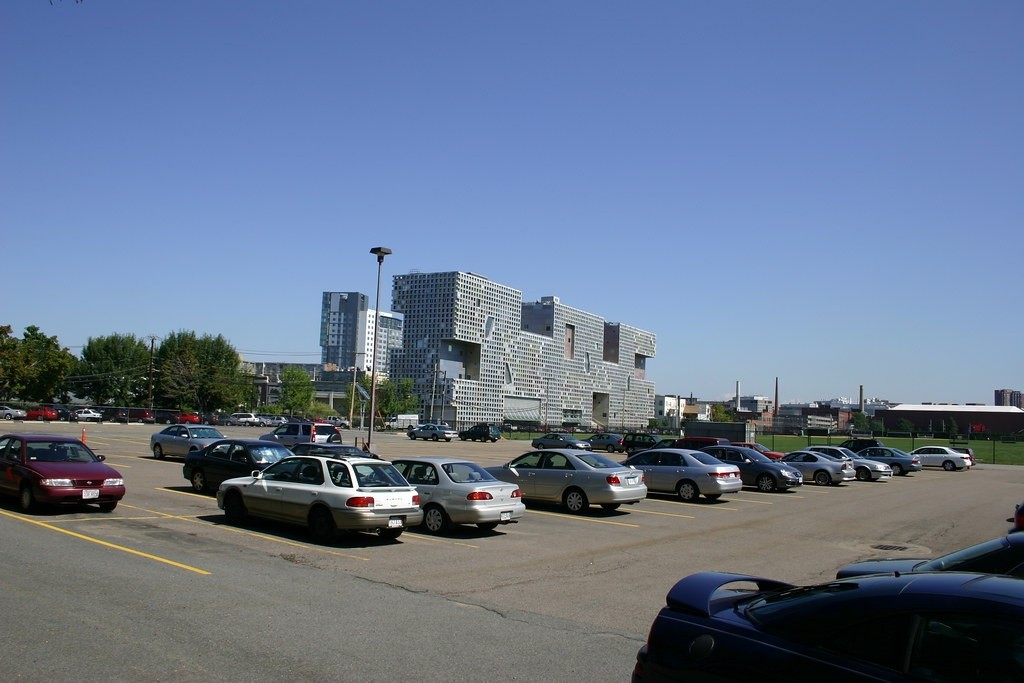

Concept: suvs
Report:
left=807, top=446, right=893, bottom=482
left=459, top=422, right=501, bottom=443
left=839, top=437, right=886, bottom=452
left=259, top=422, right=342, bottom=450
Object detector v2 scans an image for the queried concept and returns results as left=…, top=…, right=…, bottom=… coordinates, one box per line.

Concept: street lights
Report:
left=543, top=376, right=556, bottom=435
left=367, top=246, right=393, bottom=450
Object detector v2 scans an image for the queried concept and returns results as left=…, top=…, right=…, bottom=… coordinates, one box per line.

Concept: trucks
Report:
left=382, top=414, right=419, bottom=431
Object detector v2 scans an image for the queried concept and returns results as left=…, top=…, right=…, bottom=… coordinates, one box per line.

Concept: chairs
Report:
left=470, top=472, right=481, bottom=480
left=302, top=466, right=318, bottom=482
left=448, top=472, right=462, bottom=482
left=414, top=467, right=427, bottom=481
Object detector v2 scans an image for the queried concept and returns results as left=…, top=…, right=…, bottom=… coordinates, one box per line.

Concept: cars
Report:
left=697, top=442, right=857, bottom=493
left=148, top=423, right=382, bottom=495
left=387, top=457, right=526, bottom=536
left=0, top=432, right=125, bottom=514
left=630, top=501, right=1024, bottom=683
left=907, top=445, right=977, bottom=472
left=217, top=455, right=423, bottom=543
left=531, top=432, right=730, bottom=453
left=406, top=424, right=459, bottom=442
left=0, top=404, right=349, bottom=426
left=855, top=447, right=921, bottom=476
left=482, top=449, right=647, bottom=515
left=617, top=448, right=743, bottom=504
